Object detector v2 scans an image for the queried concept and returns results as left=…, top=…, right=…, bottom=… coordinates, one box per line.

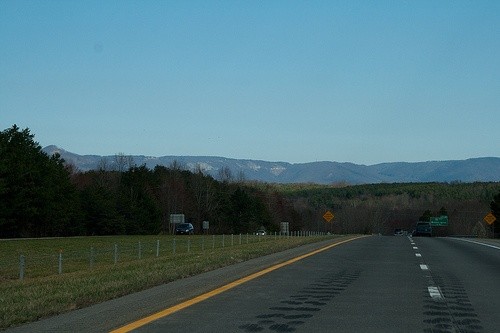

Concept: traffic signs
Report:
left=430, top=215, right=447, bottom=226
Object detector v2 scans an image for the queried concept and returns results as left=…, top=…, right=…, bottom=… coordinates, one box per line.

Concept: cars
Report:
left=255, top=230, right=266, bottom=236
left=394, top=222, right=432, bottom=238
left=175, top=222, right=194, bottom=235
